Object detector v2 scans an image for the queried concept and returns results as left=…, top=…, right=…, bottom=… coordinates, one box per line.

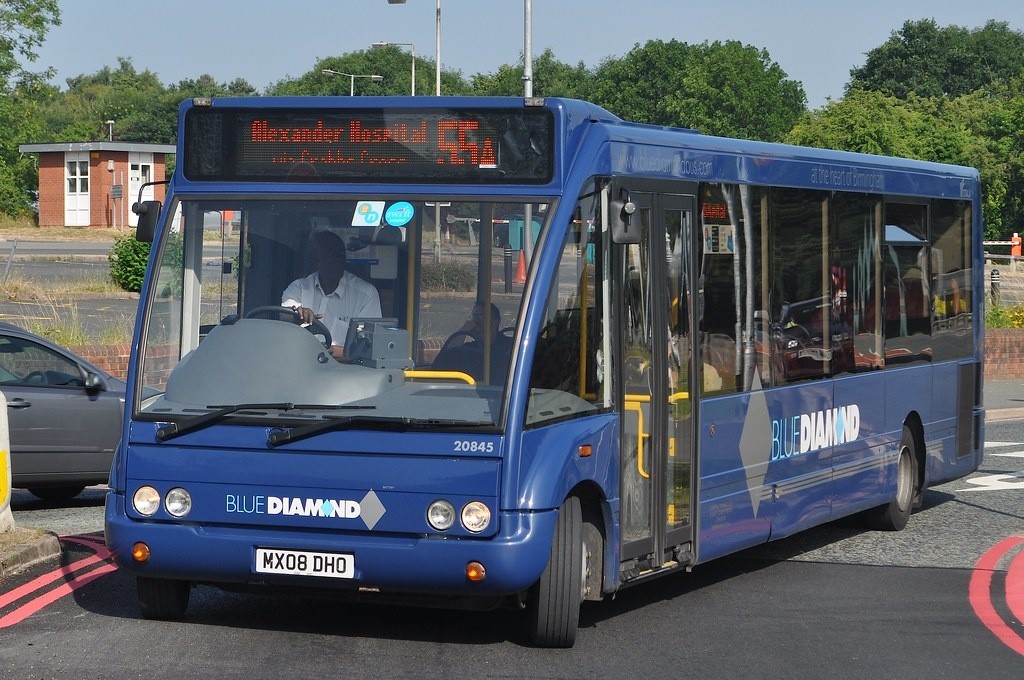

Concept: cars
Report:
left=0, top=320, right=165, bottom=501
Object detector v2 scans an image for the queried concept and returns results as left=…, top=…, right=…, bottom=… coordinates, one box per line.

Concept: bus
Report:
left=102, top=93, right=988, bottom=650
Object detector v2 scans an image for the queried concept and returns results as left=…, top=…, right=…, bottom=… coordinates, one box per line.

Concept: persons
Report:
left=933, top=269, right=966, bottom=319
left=279, top=231, right=383, bottom=363
left=447, top=302, right=512, bottom=386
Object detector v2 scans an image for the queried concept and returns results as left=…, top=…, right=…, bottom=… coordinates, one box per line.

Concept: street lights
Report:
left=387, top=0, right=442, bottom=96
left=320, top=69, right=383, bottom=97
left=105, top=119, right=115, bottom=141
left=372, top=41, right=415, bottom=96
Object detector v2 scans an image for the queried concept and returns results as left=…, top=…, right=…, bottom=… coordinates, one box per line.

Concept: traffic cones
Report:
left=443, top=225, right=451, bottom=243
left=511, top=248, right=527, bottom=284
